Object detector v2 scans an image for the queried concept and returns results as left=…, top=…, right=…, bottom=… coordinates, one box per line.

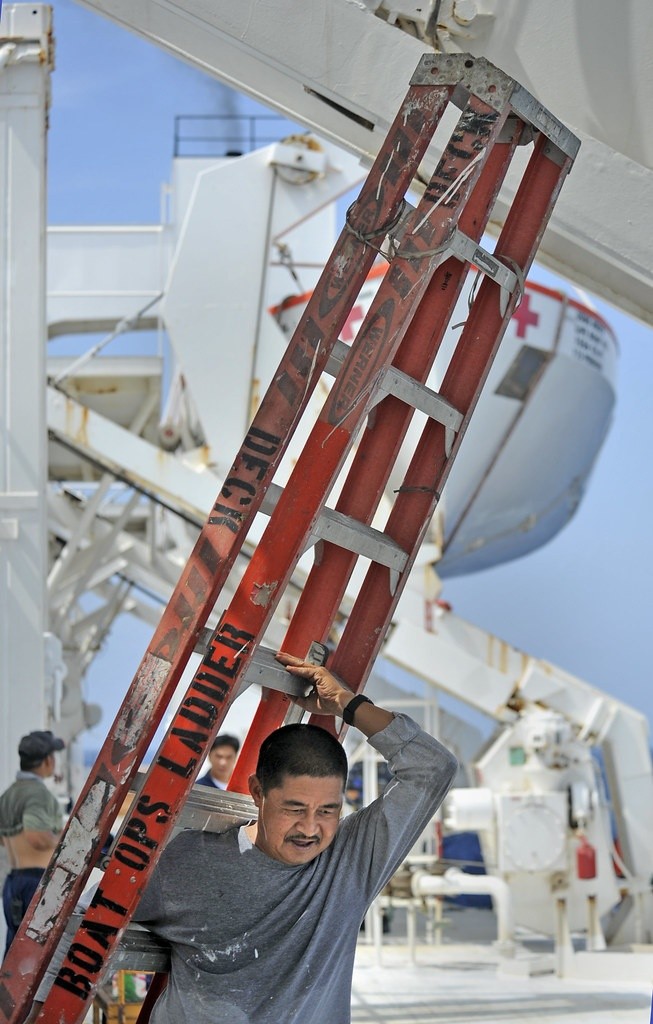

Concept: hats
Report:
left=18, top=732, right=65, bottom=758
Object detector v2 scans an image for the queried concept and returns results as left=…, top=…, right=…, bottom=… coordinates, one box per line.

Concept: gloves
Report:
left=275, top=651, right=374, bottom=727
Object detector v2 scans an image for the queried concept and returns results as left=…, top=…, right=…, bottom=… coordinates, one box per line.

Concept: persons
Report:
left=129, top=650, right=458, bottom=1024
left=0, top=729, right=72, bottom=954
left=192, top=733, right=239, bottom=794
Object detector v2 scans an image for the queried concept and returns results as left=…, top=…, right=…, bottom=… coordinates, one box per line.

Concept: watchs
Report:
left=343, top=693, right=374, bottom=727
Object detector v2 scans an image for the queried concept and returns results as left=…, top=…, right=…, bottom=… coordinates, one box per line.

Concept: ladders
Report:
left=0, top=51, right=581, bottom=1024
